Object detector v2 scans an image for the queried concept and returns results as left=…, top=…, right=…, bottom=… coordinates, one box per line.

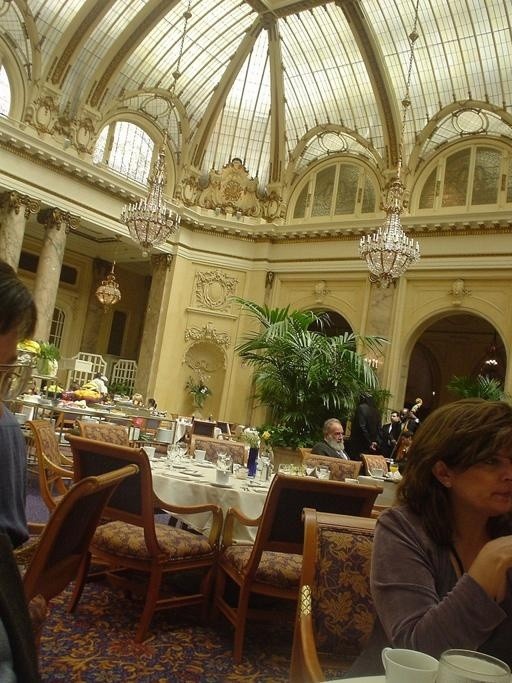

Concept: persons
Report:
left=310, top=391, right=422, bottom=476
left=341, top=399, right=512, bottom=679
left=0, top=257, right=39, bottom=683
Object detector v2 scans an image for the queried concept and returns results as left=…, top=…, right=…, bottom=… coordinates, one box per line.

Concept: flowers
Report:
left=185, top=371, right=210, bottom=409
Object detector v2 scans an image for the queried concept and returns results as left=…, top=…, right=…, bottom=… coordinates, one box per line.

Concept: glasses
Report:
left=0, top=363, right=33, bottom=403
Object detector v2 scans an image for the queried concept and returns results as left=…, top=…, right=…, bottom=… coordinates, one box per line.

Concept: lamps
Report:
left=359, top=1, right=423, bottom=288
left=96, top=234, right=123, bottom=314
left=479, top=331, right=504, bottom=371
left=121, top=0, right=192, bottom=257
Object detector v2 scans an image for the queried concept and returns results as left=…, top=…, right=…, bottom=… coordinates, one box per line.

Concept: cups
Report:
left=139, top=446, right=155, bottom=459
left=261, top=451, right=273, bottom=465
left=376, top=469, right=383, bottom=477
left=278, top=463, right=306, bottom=478
left=238, top=467, right=249, bottom=480
left=215, top=469, right=230, bottom=484
left=194, top=449, right=206, bottom=461
left=381, top=646, right=510, bottom=683
left=344, top=478, right=359, bottom=485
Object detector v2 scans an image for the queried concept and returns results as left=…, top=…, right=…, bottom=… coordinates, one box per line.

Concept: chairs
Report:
left=63, top=433, right=224, bottom=643
left=288, top=507, right=393, bottom=680
left=210, top=473, right=385, bottom=662
left=1, top=393, right=396, bottom=483
left=13, top=418, right=75, bottom=558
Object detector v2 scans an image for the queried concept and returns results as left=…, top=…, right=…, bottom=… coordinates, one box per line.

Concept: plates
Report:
left=211, top=481, right=233, bottom=489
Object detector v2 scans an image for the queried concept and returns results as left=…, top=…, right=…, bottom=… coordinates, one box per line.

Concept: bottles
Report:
left=261, top=463, right=271, bottom=482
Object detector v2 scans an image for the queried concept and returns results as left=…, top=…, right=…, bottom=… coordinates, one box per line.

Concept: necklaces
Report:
left=450, top=542, right=499, bottom=603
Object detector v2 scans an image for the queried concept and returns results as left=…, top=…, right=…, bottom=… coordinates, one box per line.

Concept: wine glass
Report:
left=215, top=445, right=232, bottom=470
left=390, top=463, right=398, bottom=477
left=303, top=459, right=329, bottom=481
left=168, top=443, right=189, bottom=469
